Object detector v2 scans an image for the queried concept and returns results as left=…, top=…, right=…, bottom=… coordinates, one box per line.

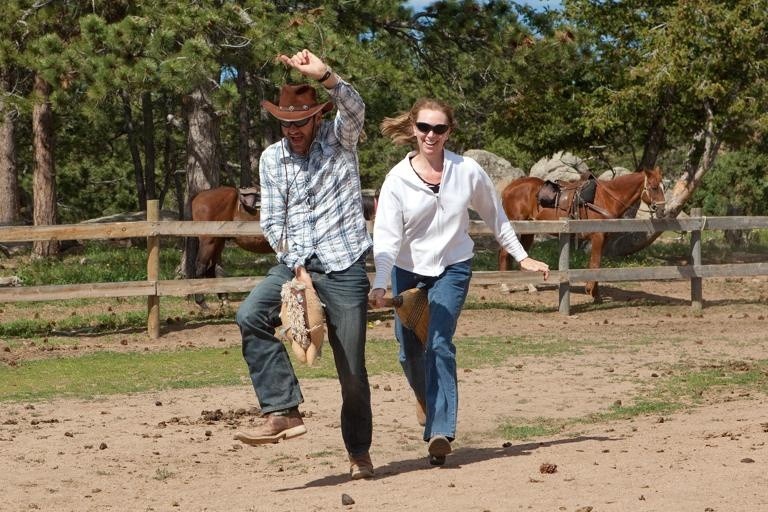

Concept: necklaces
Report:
left=410, top=157, right=440, bottom=193
left=275, top=137, right=307, bottom=256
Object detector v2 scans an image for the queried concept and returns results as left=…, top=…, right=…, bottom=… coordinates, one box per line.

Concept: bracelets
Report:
left=316, top=64, right=332, bottom=86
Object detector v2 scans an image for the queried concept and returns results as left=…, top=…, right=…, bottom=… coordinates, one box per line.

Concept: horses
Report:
left=184, top=181, right=385, bottom=315
left=500, top=166, right=667, bottom=305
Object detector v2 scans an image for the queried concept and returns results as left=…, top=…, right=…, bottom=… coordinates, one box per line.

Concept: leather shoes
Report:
left=415, top=400, right=427, bottom=426
left=234, top=409, right=307, bottom=445
left=350, top=453, right=373, bottom=478
left=428, top=435, right=452, bottom=465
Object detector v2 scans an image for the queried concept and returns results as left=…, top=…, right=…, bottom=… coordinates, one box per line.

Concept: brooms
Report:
left=365, top=286, right=429, bottom=347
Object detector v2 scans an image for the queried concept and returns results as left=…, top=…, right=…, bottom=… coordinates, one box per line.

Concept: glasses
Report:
left=279, top=118, right=312, bottom=128
left=413, top=121, right=450, bottom=135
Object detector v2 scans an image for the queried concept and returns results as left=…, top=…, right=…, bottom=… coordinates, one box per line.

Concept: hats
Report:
left=259, top=83, right=334, bottom=122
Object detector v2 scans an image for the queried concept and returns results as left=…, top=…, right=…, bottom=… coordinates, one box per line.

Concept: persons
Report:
left=367, top=98, right=551, bottom=466
left=238, top=49, right=375, bottom=479
